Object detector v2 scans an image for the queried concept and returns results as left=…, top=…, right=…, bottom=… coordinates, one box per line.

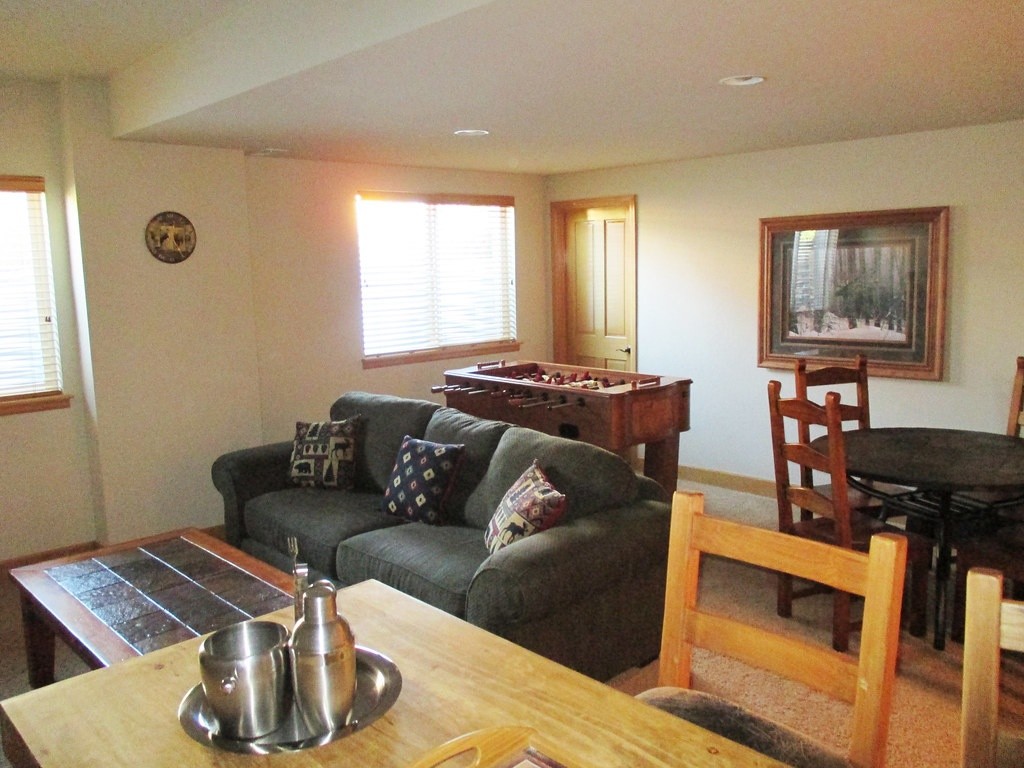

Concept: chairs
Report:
left=795, top=355, right=936, bottom=570
left=767, top=381, right=934, bottom=651
left=632, top=490, right=908, bottom=768
left=962, top=568, right=1024, bottom=768
left=951, top=356, right=1024, bottom=646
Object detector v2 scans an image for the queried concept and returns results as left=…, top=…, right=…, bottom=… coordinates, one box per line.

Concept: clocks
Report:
left=145, top=212, right=196, bottom=263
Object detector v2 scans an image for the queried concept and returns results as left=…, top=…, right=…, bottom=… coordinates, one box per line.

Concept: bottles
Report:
left=288, top=579, right=356, bottom=737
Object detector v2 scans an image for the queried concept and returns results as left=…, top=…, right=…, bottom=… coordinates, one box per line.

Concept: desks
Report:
left=809, top=428, right=1024, bottom=650
left=0, top=578, right=795, bottom=768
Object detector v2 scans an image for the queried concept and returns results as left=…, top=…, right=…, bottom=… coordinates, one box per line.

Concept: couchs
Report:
left=212, top=390, right=671, bottom=682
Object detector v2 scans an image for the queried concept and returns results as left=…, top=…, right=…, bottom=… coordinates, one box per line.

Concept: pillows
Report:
left=287, top=413, right=363, bottom=492
left=380, top=435, right=466, bottom=527
left=484, top=459, right=566, bottom=556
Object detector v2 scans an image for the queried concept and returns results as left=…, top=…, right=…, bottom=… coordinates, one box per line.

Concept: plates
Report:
left=179, top=646, right=403, bottom=754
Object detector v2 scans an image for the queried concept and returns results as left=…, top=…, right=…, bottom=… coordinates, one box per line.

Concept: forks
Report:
left=287, top=536, right=299, bottom=576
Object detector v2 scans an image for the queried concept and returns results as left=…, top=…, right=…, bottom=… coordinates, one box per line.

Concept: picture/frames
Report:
left=758, top=204, right=951, bottom=382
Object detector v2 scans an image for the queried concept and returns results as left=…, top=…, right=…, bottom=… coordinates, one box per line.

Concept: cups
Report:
left=198, top=620, right=293, bottom=738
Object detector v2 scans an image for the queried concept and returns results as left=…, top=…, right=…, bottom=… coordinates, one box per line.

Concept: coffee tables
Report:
left=9, top=527, right=308, bottom=686
